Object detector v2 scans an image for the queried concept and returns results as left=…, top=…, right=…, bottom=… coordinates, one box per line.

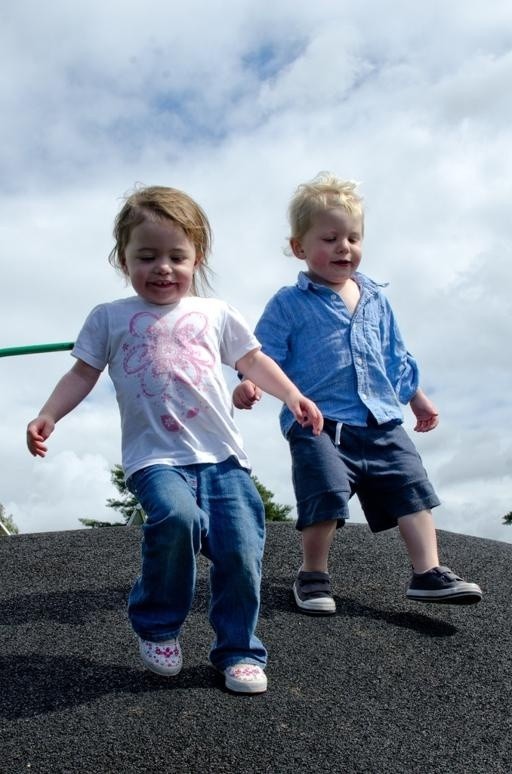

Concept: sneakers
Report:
left=140, top=639, right=183, bottom=677
left=294, top=566, right=336, bottom=614
left=223, top=663, right=268, bottom=693
left=406, top=565, right=482, bottom=604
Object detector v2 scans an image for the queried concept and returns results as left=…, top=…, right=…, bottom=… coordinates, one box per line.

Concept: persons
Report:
left=233, top=169, right=485, bottom=615
left=26, top=186, right=326, bottom=696
